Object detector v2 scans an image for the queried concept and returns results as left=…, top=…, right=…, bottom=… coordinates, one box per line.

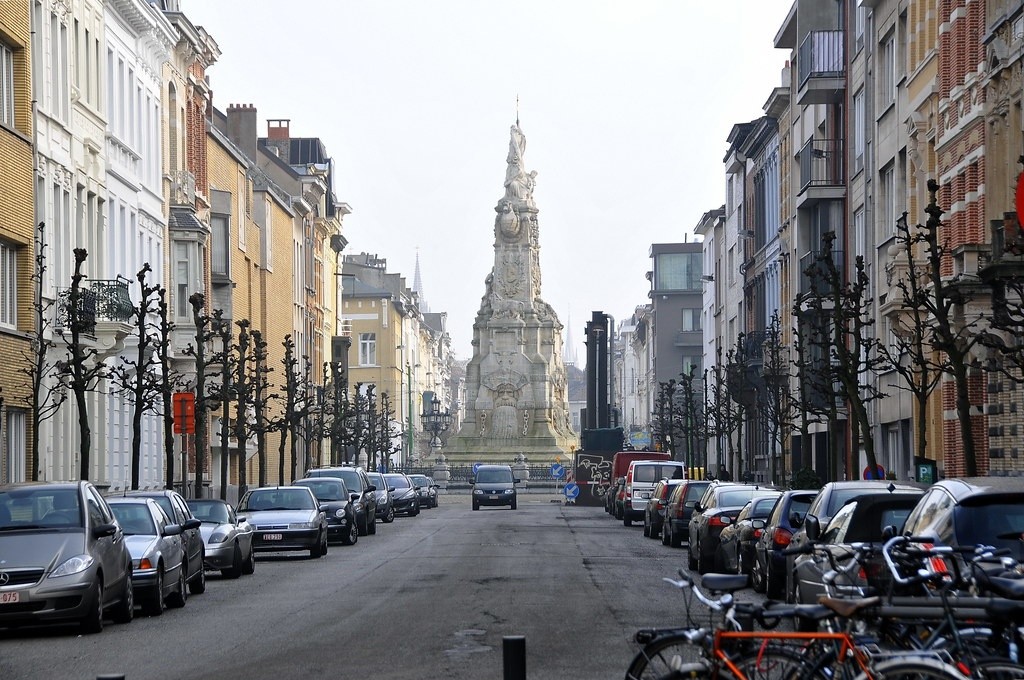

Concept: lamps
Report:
left=699, top=276, right=715, bottom=283
left=737, top=230, right=754, bottom=239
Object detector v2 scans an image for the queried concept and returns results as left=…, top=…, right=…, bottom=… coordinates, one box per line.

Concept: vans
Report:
left=617, top=460, right=685, bottom=527
left=606, top=451, right=670, bottom=518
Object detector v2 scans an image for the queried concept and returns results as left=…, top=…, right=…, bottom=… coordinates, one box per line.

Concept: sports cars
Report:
left=186, top=498, right=260, bottom=579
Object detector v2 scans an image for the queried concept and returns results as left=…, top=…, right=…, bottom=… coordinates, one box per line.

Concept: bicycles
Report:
left=625, top=523, right=1024, bottom=680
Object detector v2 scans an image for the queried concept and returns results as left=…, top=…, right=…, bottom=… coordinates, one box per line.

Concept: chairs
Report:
left=209, top=508, right=223, bottom=517
left=292, top=498, right=306, bottom=507
left=257, top=500, right=271, bottom=508
left=329, top=484, right=338, bottom=496
left=45, top=493, right=76, bottom=521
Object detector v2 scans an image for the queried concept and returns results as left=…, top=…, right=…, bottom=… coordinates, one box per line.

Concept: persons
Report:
left=714, top=464, right=733, bottom=482
left=506, top=124, right=526, bottom=181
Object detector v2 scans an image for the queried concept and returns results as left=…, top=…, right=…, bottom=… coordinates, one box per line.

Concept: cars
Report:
left=750, top=490, right=822, bottom=601
left=383, top=471, right=420, bottom=516
left=782, top=481, right=932, bottom=602
left=1, top=478, right=135, bottom=636
left=365, top=472, right=395, bottom=523
left=233, top=485, right=331, bottom=559
left=715, top=495, right=779, bottom=576
left=405, top=473, right=440, bottom=509
left=40, top=497, right=186, bottom=619
left=287, top=477, right=359, bottom=545
left=688, top=479, right=781, bottom=576
left=101, top=488, right=206, bottom=609
left=604, top=487, right=611, bottom=514
left=885, top=475, right=1023, bottom=598
left=791, top=488, right=929, bottom=630
left=612, top=476, right=627, bottom=520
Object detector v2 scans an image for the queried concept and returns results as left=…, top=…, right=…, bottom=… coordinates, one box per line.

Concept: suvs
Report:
left=469, top=465, right=521, bottom=510
left=657, top=481, right=713, bottom=548
left=302, top=467, right=378, bottom=537
left=641, top=476, right=687, bottom=539
